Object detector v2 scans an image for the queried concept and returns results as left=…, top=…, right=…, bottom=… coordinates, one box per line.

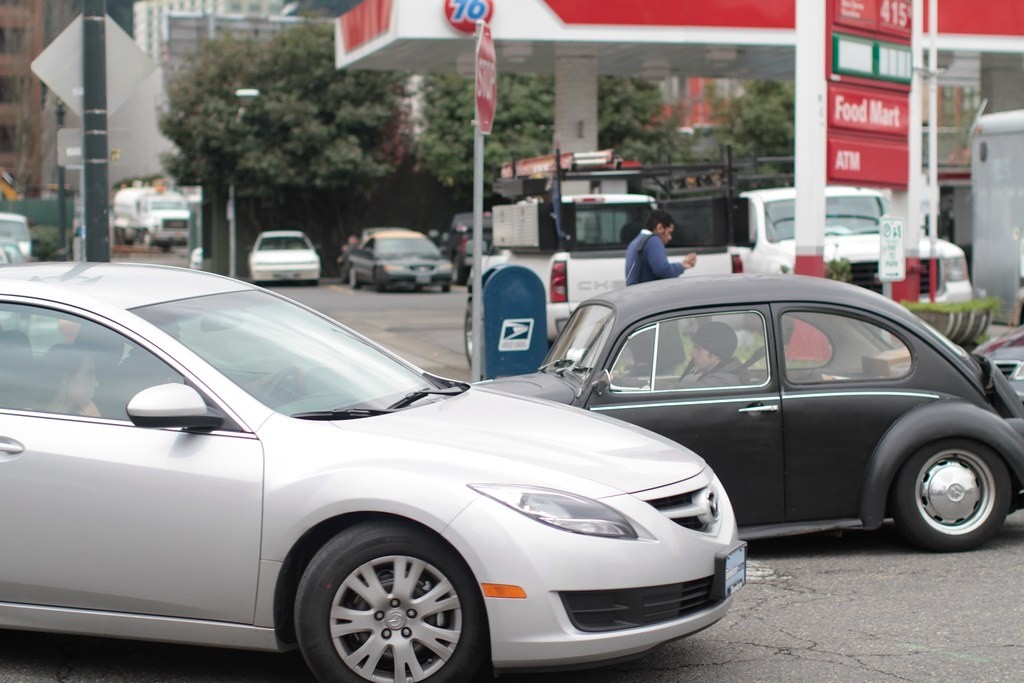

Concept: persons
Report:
left=112, top=309, right=284, bottom=430
left=26, top=342, right=101, bottom=417
left=610, top=322, right=751, bottom=391
left=625, top=210, right=696, bottom=373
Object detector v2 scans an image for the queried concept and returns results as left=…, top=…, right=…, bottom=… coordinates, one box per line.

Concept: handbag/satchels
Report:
left=634, top=233, right=662, bottom=284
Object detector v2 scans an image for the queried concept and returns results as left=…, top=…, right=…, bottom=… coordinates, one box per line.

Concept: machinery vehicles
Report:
left=112, top=180, right=192, bottom=251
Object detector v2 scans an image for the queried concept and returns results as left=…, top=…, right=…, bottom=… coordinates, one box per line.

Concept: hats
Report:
left=691, top=322, right=738, bottom=361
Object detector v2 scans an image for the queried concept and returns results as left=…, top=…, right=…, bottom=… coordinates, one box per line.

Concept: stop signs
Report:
left=475, top=23, right=497, bottom=135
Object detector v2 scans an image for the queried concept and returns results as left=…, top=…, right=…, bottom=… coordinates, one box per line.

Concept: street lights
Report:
left=226, top=88, right=260, bottom=279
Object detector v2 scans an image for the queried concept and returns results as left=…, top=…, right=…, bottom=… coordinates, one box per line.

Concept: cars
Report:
left=344, top=227, right=453, bottom=294
left=0, top=213, right=32, bottom=261
left=464, top=186, right=973, bottom=368
left=0, top=262, right=748, bottom=683
left=970, top=324, right=1024, bottom=403
left=249, top=231, right=320, bottom=287
left=471, top=273, right=1024, bottom=553
